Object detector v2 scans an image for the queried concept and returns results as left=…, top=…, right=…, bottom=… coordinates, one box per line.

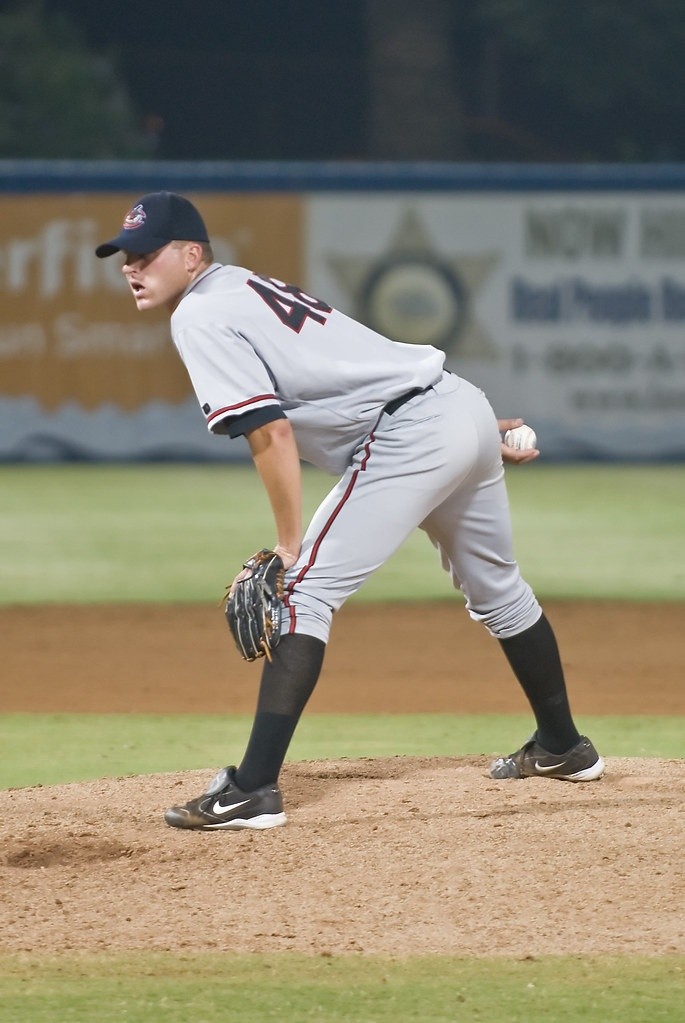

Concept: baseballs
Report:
left=504, top=424, right=536, bottom=451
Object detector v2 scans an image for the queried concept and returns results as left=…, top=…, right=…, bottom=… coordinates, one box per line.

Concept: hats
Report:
left=93, top=190, right=209, bottom=258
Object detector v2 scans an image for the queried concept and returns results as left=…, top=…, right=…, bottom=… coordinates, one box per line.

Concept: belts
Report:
left=383, top=384, right=433, bottom=416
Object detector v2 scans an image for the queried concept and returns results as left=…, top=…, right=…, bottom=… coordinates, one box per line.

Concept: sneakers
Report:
left=505, top=729, right=604, bottom=782
left=164, top=765, right=286, bottom=831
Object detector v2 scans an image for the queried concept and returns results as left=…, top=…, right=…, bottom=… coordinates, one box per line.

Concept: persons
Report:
left=95, top=189, right=606, bottom=831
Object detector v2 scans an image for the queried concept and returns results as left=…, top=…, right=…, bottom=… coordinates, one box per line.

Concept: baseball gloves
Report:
left=220, top=548, right=284, bottom=663
